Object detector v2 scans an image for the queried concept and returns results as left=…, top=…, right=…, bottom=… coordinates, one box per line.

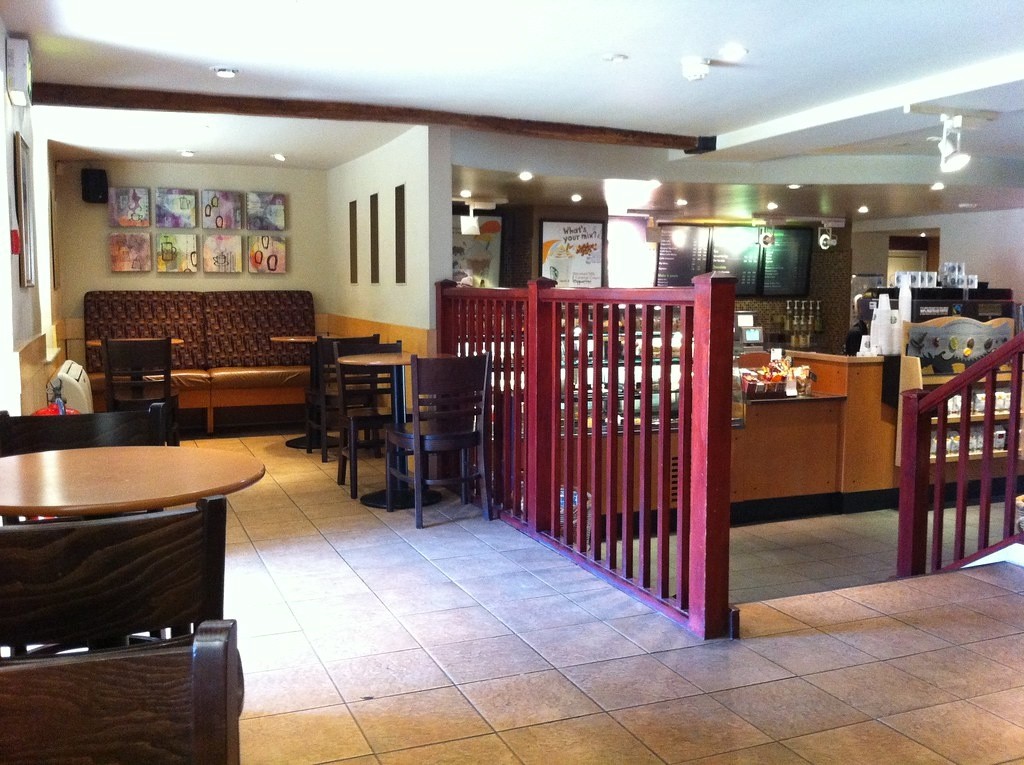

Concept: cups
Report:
left=547, top=255, right=574, bottom=288
left=466, top=255, right=492, bottom=279
left=893, top=261, right=978, bottom=288
left=858, top=274, right=913, bottom=356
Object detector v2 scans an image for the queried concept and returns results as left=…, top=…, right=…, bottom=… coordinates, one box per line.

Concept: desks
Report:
left=383, top=351, right=494, bottom=529
left=269, top=335, right=349, bottom=449
left=86, top=338, right=184, bottom=382
left=337, top=352, right=457, bottom=510
left=0, top=445, right=266, bottom=658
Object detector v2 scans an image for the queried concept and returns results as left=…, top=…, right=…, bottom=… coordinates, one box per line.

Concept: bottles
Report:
left=782, top=299, right=826, bottom=349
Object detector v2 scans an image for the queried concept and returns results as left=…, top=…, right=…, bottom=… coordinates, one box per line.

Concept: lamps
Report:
left=936, top=113, right=972, bottom=173
left=758, top=225, right=775, bottom=249
left=817, top=226, right=836, bottom=250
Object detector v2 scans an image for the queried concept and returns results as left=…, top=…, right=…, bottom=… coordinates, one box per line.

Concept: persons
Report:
left=841, top=297, right=875, bottom=356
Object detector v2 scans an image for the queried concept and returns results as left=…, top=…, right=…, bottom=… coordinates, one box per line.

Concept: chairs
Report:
left=0, top=494, right=227, bottom=659
left=333, top=339, right=414, bottom=499
left=100, top=335, right=180, bottom=447
left=304, top=333, right=382, bottom=463
left=0, top=403, right=168, bottom=458
left=0, top=619, right=245, bottom=765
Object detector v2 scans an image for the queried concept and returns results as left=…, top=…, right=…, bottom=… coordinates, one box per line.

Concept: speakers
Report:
left=81, top=168, right=109, bottom=203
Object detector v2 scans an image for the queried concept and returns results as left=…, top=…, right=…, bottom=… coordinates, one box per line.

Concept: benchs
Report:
left=84, top=290, right=316, bottom=434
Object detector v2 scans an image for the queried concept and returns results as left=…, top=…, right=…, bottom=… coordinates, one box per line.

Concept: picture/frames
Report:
left=15, top=131, right=35, bottom=288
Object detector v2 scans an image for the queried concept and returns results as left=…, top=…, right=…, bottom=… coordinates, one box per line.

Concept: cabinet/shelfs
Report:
left=894, top=315, right=1024, bottom=467
